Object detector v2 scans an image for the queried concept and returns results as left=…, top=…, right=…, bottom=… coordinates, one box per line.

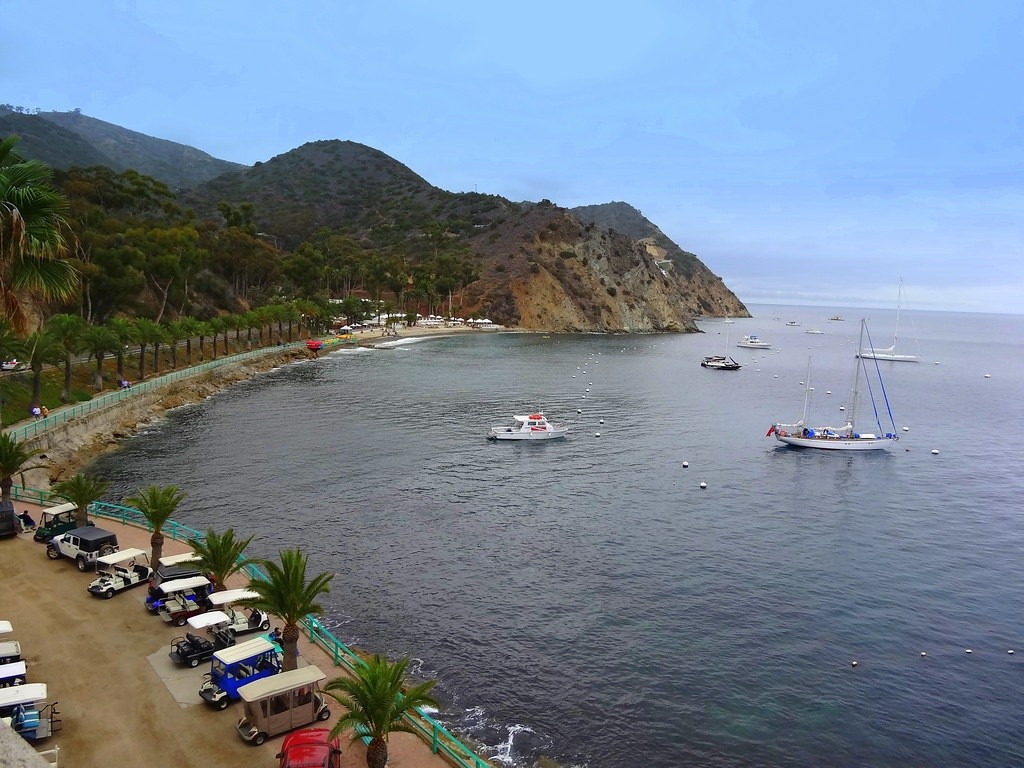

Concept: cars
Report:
left=276, top=728, right=342, bottom=768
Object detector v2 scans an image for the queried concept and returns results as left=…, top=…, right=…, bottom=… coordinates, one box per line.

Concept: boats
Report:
left=492, top=412, right=564, bottom=440
left=542, top=336, right=552, bottom=338
left=804, top=328, right=824, bottom=334
left=828, top=315, right=846, bottom=321
left=786, top=322, right=800, bottom=326
left=736, top=335, right=772, bottom=348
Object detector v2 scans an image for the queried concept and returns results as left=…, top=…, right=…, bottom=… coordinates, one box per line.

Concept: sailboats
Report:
left=774, top=318, right=899, bottom=450
left=700, top=324, right=742, bottom=370
left=856, top=272, right=921, bottom=362
left=722, top=313, right=735, bottom=324
